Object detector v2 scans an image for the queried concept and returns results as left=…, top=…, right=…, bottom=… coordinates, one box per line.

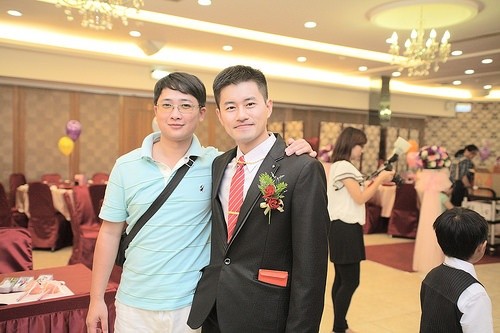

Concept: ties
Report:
left=227, top=155, right=246, bottom=243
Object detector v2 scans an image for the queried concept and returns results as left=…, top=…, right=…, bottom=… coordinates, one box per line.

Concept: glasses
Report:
left=156, top=103, right=202, bottom=113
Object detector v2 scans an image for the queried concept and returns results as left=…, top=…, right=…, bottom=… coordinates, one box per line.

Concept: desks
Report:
left=0, top=263, right=117, bottom=333
left=16, top=183, right=75, bottom=221
left=365, top=180, right=397, bottom=217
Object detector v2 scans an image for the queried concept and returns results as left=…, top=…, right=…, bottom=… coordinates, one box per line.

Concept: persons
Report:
left=187, top=64, right=329, bottom=333
left=84, top=72, right=317, bottom=333
left=327, top=127, right=395, bottom=333
left=419, top=207, right=493, bottom=333
left=449, top=144, right=479, bottom=207
left=440, top=191, right=453, bottom=213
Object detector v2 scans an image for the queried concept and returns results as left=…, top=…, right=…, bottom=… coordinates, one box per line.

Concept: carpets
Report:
left=365, top=241, right=500, bottom=273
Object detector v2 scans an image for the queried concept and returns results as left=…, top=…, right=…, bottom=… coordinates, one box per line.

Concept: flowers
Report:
left=258, top=172, right=288, bottom=225
left=414, top=145, right=452, bottom=172
left=308, top=137, right=334, bottom=162
left=476, top=147, right=490, bottom=163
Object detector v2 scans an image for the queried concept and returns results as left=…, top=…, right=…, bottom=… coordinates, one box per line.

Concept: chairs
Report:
left=362, top=203, right=382, bottom=234
left=388, top=184, right=419, bottom=238
left=0, top=173, right=109, bottom=274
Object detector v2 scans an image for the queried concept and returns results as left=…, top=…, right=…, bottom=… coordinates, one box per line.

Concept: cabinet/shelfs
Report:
left=463, top=194, right=500, bottom=256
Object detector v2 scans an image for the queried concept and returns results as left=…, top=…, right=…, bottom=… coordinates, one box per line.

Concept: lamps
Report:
left=388, top=3, right=451, bottom=76
left=53, top=0, right=144, bottom=30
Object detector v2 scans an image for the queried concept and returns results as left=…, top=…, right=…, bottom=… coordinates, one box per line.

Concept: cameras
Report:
left=384, top=154, right=405, bottom=187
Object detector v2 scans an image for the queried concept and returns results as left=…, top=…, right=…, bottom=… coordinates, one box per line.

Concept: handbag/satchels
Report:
left=115, top=225, right=129, bottom=267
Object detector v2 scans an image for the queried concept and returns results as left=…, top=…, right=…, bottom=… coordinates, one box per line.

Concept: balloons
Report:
left=58, top=120, right=81, bottom=155
